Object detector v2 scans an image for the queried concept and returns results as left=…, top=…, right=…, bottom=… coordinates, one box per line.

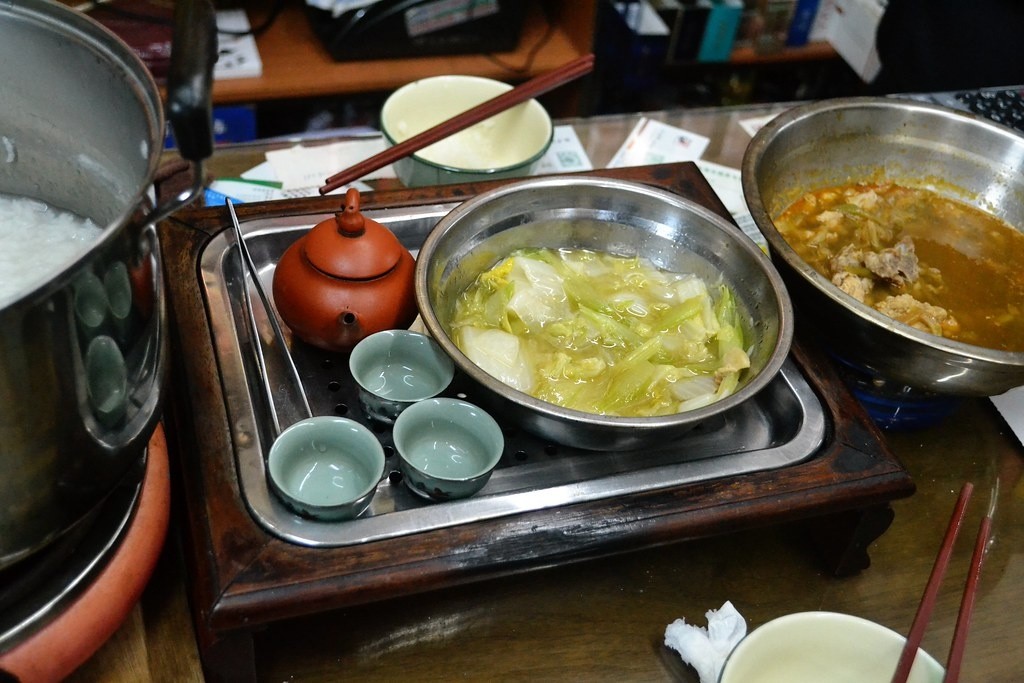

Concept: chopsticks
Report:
left=318, top=51, right=595, bottom=194
left=890, top=481, right=992, bottom=683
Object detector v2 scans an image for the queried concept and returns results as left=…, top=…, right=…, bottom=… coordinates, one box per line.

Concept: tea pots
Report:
left=271, top=186, right=421, bottom=355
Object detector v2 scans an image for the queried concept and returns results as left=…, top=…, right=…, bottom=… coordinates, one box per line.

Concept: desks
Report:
left=82, top=0, right=594, bottom=107
left=716, top=41, right=837, bottom=104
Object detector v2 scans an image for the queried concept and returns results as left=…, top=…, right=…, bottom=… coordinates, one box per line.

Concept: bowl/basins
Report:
left=379, top=72, right=556, bottom=191
left=414, top=176, right=793, bottom=455
left=742, top=95, right=1023, bottom=398
left=716, top=611, right=948, bottom=683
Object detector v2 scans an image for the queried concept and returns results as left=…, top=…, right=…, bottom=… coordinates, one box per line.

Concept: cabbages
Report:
left=449, top=245, right=751, bottom=416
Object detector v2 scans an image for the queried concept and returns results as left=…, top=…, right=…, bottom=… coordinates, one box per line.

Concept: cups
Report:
left=267, top=414, right=387, bottom=522
left=348, top=329, right=456, bottom=424
left=392, top=396, right=506, bottom=502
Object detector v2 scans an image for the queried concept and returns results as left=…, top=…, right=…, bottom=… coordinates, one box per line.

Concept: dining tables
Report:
left=52, top=85, right=1024, bottom=683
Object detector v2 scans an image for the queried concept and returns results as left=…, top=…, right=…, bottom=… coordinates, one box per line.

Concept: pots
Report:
left=1, top=0, right=218, bottom=683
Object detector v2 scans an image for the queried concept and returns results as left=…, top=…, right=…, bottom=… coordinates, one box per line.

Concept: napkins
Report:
left=664, top=600, right=747, bottom=683
